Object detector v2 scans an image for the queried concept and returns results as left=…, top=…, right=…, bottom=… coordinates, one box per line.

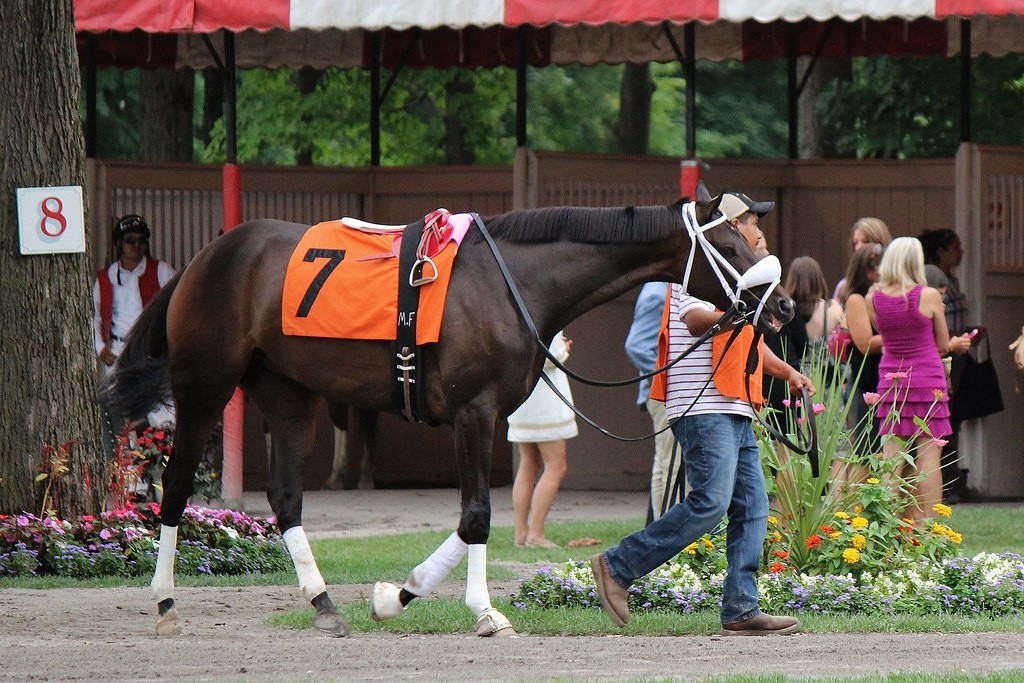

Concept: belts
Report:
left=110, top=334, right=126, bottom=342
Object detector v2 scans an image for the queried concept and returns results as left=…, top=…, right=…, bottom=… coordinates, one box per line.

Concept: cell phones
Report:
left=967, top=329, right=978, bottom=338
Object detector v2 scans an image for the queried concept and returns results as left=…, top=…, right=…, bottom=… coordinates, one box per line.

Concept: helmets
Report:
left=114, top=214, right=150, bottom=243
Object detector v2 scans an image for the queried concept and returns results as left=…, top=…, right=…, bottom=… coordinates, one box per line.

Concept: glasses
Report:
left=121, top=234, right=150, bottom=245
left=870, top=243, right=883, bottom=261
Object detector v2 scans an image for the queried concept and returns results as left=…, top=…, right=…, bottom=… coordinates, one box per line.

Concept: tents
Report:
left=71, top=2, right=1024, bottom=160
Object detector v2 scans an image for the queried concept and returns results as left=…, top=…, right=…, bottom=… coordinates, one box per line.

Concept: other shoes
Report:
left=514, top=540, right=526, bottom=550
left=146, top=465, right=163, bottom=506
left=525, top=540, right=560, bottom=549
left=721, top=612, right=802, bottom=636
left=590, top=553, right=631, bottom=629
left=941, top=485, right=982, bottom=506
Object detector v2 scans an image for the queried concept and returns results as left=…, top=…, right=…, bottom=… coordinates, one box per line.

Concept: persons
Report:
left=506, top=328, right=579, bottom=550
left=92, top=214, right=179, bottom=505
left=777, top=253, right=846, bottom=533
left=831, top=216, right=988, bottom=550
left=588, top=186, right=806, bottom=639
left=622, top=281, right=692, bottom=525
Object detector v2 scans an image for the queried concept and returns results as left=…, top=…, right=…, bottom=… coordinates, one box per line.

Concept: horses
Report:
left=98, top=180, right=796, bottom=640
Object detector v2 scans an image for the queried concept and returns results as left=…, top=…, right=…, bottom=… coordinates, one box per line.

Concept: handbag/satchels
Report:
left=950, top=326, right=1005, bottom=421
left=826, top=322, right=852, bottom=366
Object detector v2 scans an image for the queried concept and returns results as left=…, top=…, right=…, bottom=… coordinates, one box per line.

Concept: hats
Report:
left=714, top=191, right=774, bottom=221
